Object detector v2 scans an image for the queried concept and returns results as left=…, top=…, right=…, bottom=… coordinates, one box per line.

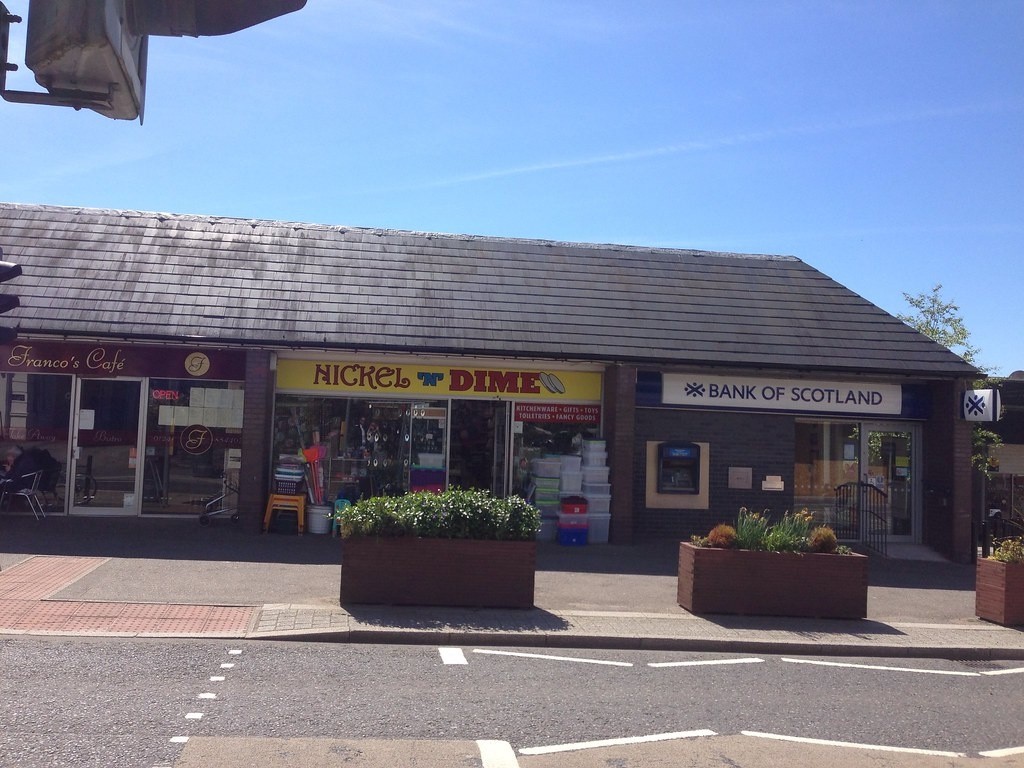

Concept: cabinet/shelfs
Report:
left=323, top=458, right=372, bottom=494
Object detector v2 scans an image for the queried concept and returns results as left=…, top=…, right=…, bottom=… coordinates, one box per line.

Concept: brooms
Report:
left=297, top=442, right=327, bottom=506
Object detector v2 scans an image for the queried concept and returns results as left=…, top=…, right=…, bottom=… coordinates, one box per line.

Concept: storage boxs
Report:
left=582, top=481, right=611, bottom=496
left=583, top=439, right=607, bottom=453
left=581, top=465, right=610, bottom=484
left=530, top=454, right=591, bottom=547
left=582, top=452, right=608, bottom=467
left=583, top=494, right=612, bottom=514
left=587, top=512, right=611, bottom=545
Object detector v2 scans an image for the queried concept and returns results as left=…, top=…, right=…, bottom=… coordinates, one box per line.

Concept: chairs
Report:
left=0, top=469, right=46, bottom=521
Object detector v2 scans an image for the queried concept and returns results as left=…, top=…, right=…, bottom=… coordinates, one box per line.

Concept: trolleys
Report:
left=197, top=471, right=241, bottom=527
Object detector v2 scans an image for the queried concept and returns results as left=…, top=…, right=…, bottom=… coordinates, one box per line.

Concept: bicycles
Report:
left=35, top=464, right=97, bottom=505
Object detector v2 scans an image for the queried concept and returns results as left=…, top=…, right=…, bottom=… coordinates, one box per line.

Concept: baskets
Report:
left=271, top=463, right=305, bottom=496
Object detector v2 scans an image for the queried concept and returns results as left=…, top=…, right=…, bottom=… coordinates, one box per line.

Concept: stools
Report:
left=262, top=492, right=308, bottom=537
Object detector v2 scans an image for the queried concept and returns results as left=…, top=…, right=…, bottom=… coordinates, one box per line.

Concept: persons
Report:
left=0, top=442, right=52, bottom=504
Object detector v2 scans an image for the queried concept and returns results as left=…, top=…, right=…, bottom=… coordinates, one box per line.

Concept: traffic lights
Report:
left=25, top=0, right=306, bottom=127
left=0, top=249, right=23, bottom=343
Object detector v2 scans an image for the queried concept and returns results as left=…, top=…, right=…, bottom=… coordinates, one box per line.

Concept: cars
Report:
left=533, top=430, right=595, bottom=458
